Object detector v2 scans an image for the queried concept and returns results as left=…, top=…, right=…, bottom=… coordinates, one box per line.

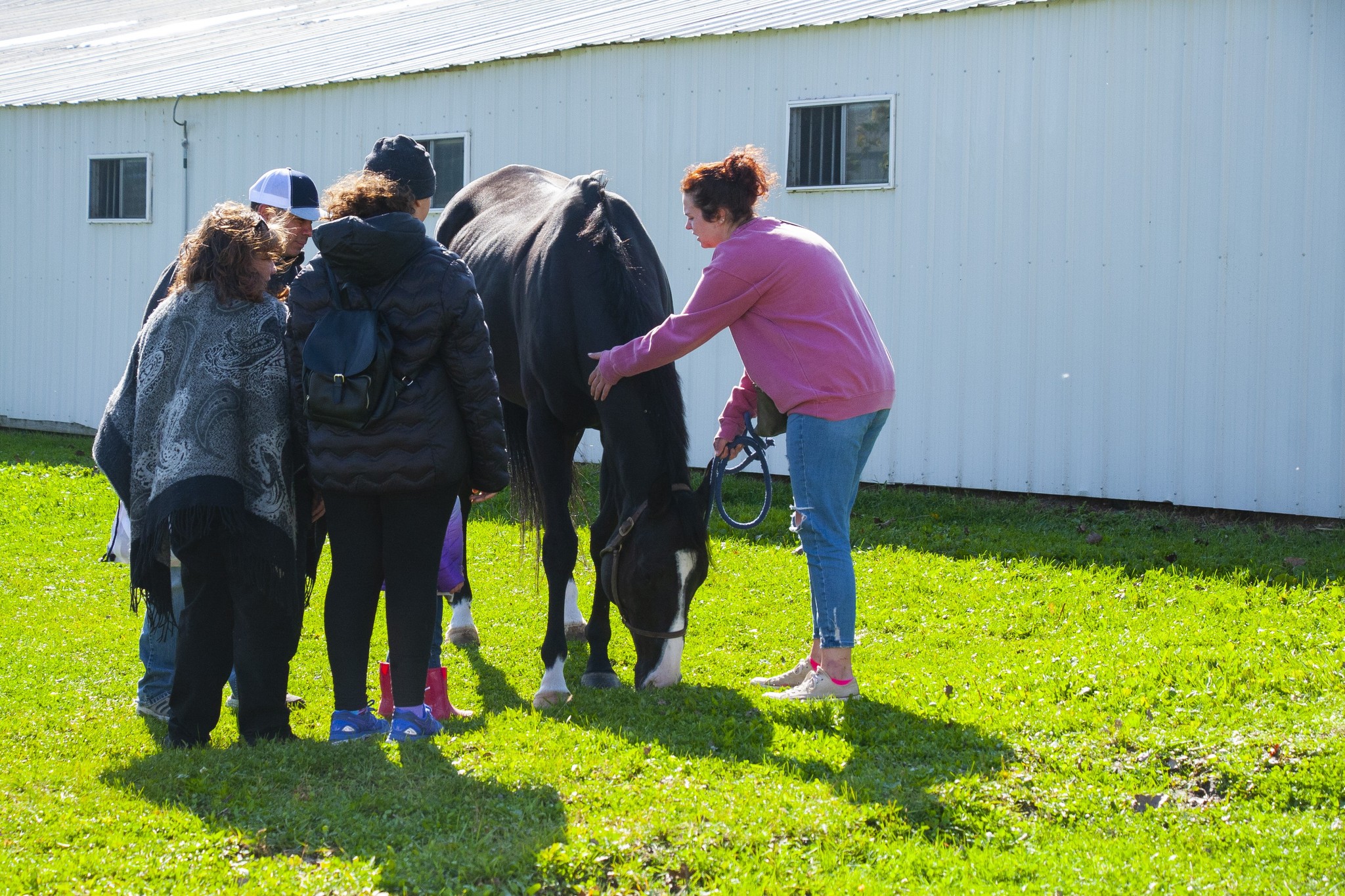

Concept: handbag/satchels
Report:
left=751, top=382, right=788, bottom=437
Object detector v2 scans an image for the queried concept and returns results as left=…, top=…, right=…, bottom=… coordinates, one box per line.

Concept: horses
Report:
left=436, top=166, right=715, bottom=717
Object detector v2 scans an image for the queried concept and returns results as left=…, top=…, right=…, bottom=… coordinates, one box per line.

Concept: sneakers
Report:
left=329, top=699, right=390, bottom=746
left=225, top=692, right=303, bottom=709
left=135, top=694, right=172, bottom=722
left=386, top=704, right=447, bottom=743
left=750, top=656, right=815, bottom=688
left=762, top=665, right=861, bottom=703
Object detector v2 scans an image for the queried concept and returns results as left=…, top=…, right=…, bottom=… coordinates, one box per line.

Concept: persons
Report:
left=283, top=132, right=512, bottom=751
left=378, top=494, right=478, bottom=726
left=589, top=144, right=899, bottom=700
left=137, top=167, right=325, bottom=726
left=103, top=200, right=308, bottom=750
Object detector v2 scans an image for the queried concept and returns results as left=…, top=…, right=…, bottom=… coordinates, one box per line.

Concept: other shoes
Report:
left=162, top=735, right=209, bottom=753
left=241, top=731, right=302, bottom=747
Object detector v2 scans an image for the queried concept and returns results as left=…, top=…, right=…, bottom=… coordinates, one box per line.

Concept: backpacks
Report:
left=301, top=247, right=450, bottom=432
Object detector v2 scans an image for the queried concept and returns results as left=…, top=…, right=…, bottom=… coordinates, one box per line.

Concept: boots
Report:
left=378, top=662, right=394, bottom=716
left=424, top=667, right=474, bottom=721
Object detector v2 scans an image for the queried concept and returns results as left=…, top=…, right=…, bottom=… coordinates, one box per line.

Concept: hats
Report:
left=249, top=167, right=333, bottom=221
left=362, top=134, right=436, bottom=200
